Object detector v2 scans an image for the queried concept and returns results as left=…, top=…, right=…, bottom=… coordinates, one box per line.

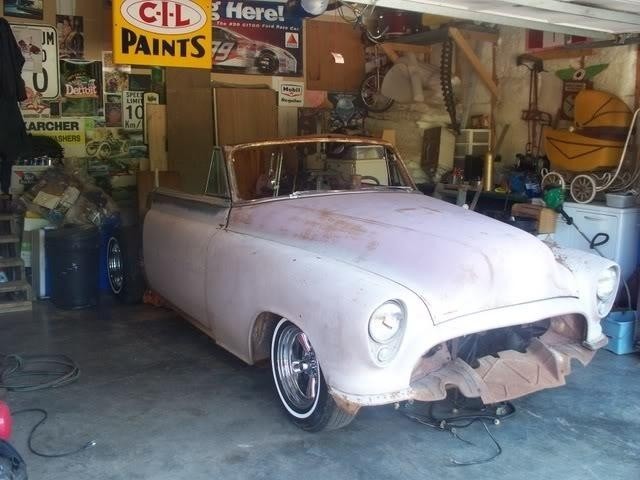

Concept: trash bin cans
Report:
left=66, top=212, right=121, bottom=289
left=48, top=225, right=99, bottom=309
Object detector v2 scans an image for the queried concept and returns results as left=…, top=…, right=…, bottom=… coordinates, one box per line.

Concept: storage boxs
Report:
left=605, top=191, right=636, bottom=209
left=509, top=203, right=557, bottom=234
left=599, top=310, right=640, bottom=355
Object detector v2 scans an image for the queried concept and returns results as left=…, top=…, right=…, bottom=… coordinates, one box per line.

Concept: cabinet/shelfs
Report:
left=454, top=129, right=491, bottom=169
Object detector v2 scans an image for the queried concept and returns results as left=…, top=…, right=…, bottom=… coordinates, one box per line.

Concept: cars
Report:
left=102, top=135, right=622, bottom=435
